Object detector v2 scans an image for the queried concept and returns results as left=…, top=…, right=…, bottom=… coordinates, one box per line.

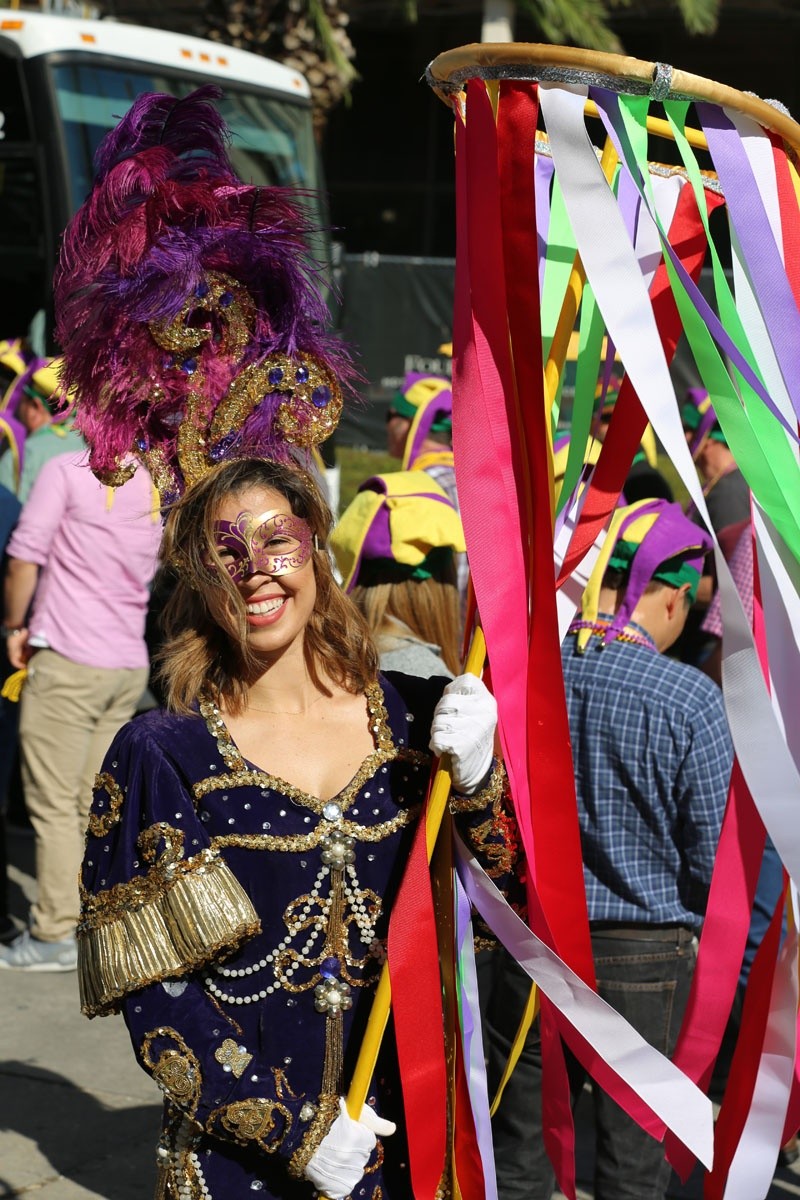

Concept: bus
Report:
left=0, top=7, right=337, bottom=416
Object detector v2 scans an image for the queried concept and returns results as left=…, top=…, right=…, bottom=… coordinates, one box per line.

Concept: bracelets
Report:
left=4, top=624, right=23, bottom=638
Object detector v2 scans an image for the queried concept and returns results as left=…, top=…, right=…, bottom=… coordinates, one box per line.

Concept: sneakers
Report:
left=0, top=930, right=78, bottom=973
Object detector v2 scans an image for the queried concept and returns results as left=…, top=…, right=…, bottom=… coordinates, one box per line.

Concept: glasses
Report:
left=384, top=409, right=402, bottom=426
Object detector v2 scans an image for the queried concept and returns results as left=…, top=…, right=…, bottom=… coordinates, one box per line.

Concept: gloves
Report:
left=429, top=673, right=499, bottom=795
left=303, top=1093, right=396, bottom=1200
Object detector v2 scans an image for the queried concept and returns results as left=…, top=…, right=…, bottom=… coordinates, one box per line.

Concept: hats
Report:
left=679, top=384, right=730, bottom=462
left=324, top=471, right=467, bottom=596
left=554, top=428, right=604, bottom=480
left=0, top=337, right=77, bottom=404
left=590, top=374, right=659, bottom=471
left=392, top=370, right=456, bottom=472
left=576, top=495, right=714, bottom=654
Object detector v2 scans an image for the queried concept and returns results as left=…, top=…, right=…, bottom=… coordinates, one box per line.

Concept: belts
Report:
left=589, top=920, right=695, bottom=941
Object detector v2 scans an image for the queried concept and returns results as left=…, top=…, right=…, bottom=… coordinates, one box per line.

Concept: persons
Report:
left=0, top=355, right=163, bottom=972
left=336, top=370, right=800, bottom=1200
left=76, top=449, right=527, bottom=1200
